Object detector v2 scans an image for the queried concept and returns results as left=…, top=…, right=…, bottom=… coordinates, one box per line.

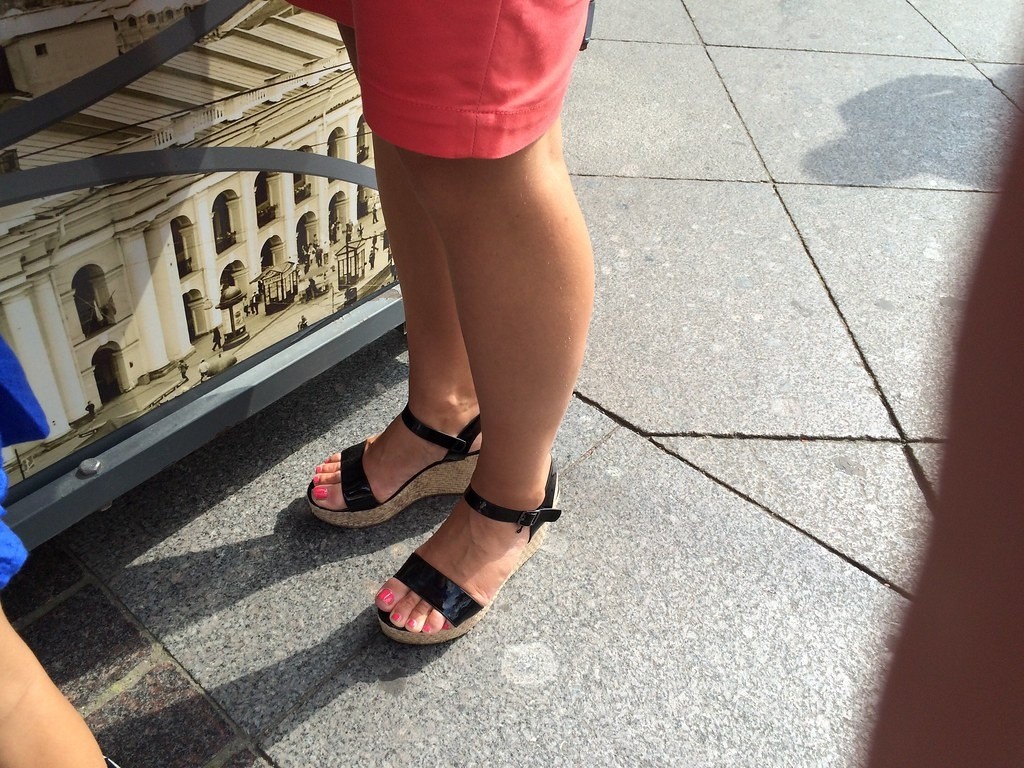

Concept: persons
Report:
left=286, top=0, right=596, bottom=644
left=0, top=334, right=121, bottom=768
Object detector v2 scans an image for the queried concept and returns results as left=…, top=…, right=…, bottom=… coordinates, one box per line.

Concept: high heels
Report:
left=374, top=456, right=561, bottom=644
left=306, top=404, right=483, bottom=528
